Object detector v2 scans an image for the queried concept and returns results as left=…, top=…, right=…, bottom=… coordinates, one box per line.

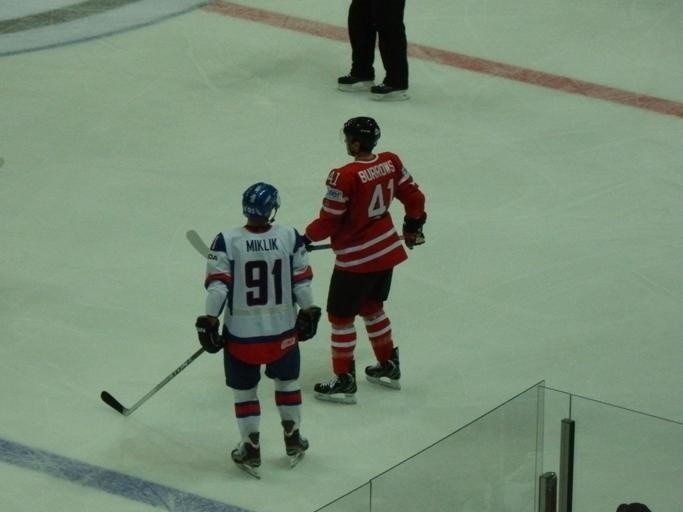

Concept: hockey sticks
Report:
left=100, top=346, right=207, bottom=416
left=186, top=230, right=406, bottom=257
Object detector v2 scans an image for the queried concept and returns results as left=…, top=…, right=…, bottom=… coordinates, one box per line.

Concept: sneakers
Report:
left=370, top=80, right=409, bottom=94
left=280, top=419, right=310, bottom=457
left=364, top=346, right=402, bottom=381
left=314, top=360, right=358, bottom=396
left=337, top=64, right=375, bottom=84
left=230, top=432, right=262, bottom=467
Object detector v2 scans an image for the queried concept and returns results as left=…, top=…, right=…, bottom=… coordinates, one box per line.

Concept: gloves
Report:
left=295, top=305, right=321, bottom=342
left=403, top=213, right=427, bottom=251
left=195, top=314, right=226, bottom=354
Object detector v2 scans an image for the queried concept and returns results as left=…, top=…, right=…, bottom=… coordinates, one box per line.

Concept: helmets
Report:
left=241, top=182, right=282, bottom=224
left=343, top=116, right=381, bottom=151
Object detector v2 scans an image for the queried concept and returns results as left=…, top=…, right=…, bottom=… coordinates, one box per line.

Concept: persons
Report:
left=338, top=1, right=409, bottom=92
left=195, top=182, right=321, bottom=464
left=302, top=116, right=429, bottom=395
